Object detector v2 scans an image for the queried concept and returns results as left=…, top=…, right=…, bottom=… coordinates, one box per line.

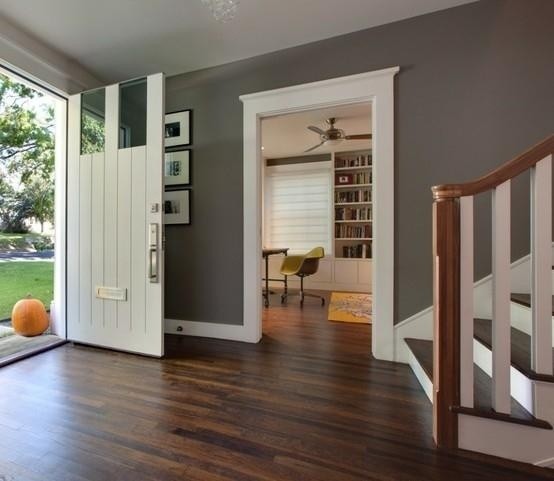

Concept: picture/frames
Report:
left=164, top=108, right=194, bottom=227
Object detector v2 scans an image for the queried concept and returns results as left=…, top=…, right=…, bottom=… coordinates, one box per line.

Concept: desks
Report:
left=261, top=247, right=290, bottom=307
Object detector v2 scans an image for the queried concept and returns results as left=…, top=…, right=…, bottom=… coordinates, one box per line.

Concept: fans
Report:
left=303, top=117, right=372, bottom=154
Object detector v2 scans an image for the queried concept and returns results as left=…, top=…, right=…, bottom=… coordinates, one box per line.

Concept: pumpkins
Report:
left=11, top=298, right=50, bottom=336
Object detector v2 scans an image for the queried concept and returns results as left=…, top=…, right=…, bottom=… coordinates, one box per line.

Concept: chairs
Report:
left=279, top=247, right=325, bottom=308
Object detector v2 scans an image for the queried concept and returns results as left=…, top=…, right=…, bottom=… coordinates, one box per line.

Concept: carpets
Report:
left=328, top=292, right=372, bottom=325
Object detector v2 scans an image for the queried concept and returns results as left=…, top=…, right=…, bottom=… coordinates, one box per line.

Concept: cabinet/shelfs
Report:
left=331, top=147, right=373, bottom=292
